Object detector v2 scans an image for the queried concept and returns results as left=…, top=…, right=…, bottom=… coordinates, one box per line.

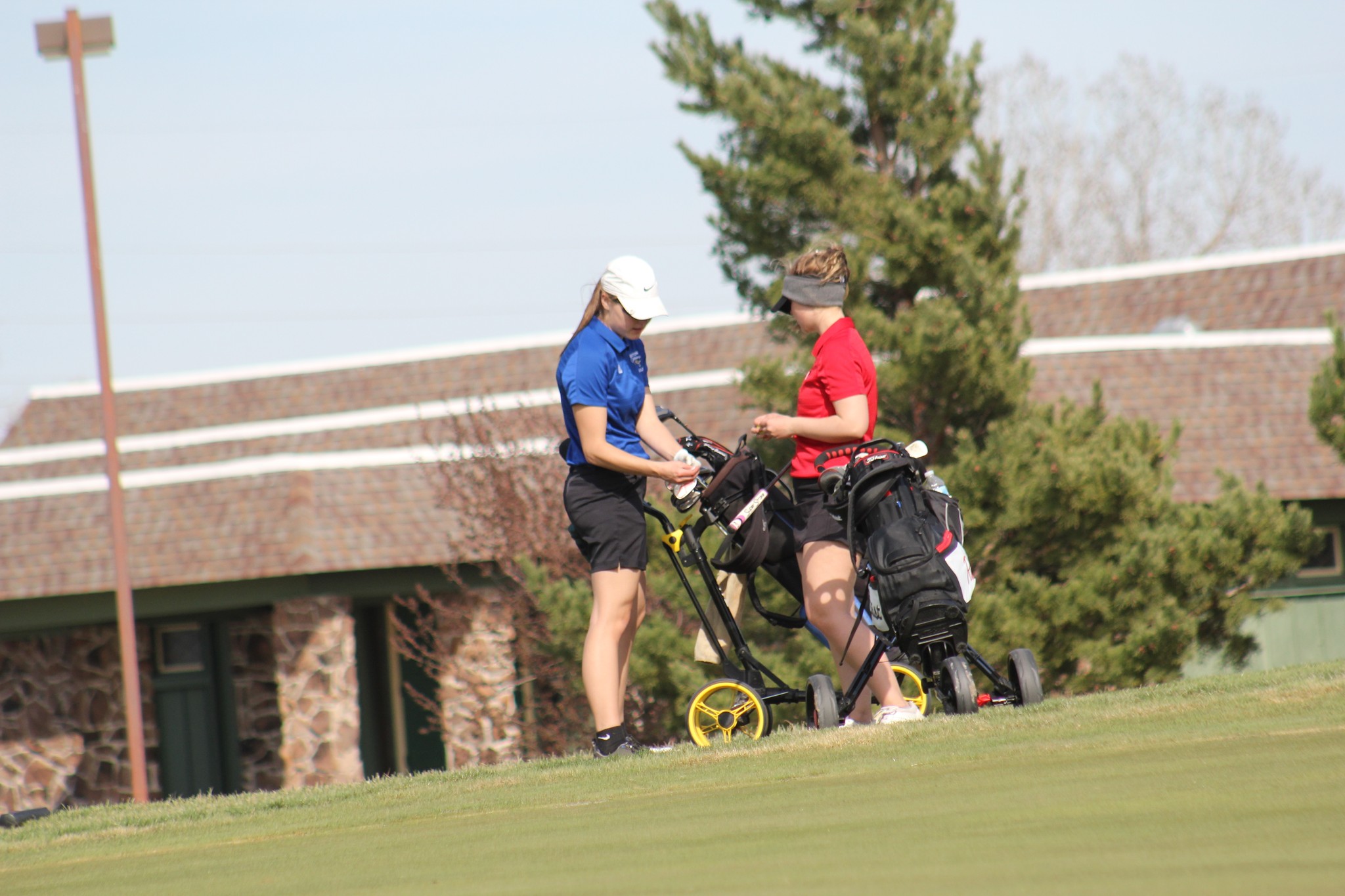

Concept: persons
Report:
left=751, top=245, right=926, bottom=729
left=555, top=257, right=701, bottom=761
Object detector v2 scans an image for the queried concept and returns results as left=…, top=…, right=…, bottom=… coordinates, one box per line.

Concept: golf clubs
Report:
left=657, top=409, right=726, bottom=464
left=664, top=479, right=710, bottom=514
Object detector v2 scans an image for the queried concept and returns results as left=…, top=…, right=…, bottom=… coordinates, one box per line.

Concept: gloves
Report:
left=665, top=449, right=701, bottom=500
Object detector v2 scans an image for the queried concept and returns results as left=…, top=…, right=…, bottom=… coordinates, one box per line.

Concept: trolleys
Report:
left=805, top=437, right=1045, bottom=731
left=644, top=435, right=935, bottom=748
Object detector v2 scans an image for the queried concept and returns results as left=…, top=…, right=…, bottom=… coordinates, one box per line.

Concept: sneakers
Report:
left=626, top=734, right=672, bottom=753
left=871, top=701, right=924, bottom=725
left=591, top=738, right=642, bottom=760
left=838, top=716, right=860, bottom=728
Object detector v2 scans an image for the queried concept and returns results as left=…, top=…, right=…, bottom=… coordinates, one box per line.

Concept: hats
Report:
left=601, top=255, right=669, bottom=320
left=771, top=275, right=846, bottom=316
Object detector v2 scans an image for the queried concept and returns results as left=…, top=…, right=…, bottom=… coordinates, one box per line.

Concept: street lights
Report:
left=34, top=8, right=149, bottom=803
